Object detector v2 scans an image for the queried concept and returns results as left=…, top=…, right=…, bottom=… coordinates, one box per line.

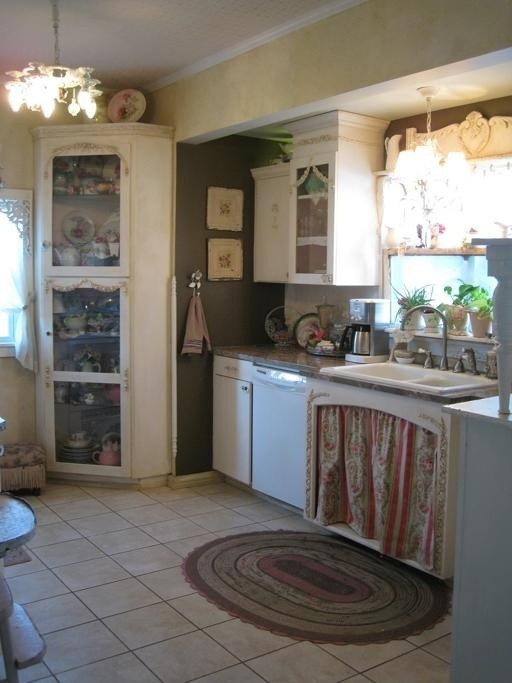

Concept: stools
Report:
left=2, top=442, right=45, bottom=496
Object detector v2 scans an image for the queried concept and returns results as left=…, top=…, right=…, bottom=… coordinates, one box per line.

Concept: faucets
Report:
left=461, top=347, right=477, bottom=372
left=401, top=305, right=449, bottom=371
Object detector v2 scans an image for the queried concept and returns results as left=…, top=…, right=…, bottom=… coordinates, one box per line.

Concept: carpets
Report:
left=181, top=529, right=452, bottom=646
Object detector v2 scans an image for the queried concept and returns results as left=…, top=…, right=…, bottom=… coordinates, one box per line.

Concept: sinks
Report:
left=319, top=361, right=437, bottom=385
left=407, top=371, right=499, bottom=396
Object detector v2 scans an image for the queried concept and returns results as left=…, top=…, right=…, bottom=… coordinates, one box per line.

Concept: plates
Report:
left=61, top=211, right=96, bottom=243
left=293, top=312, right=324, bottom=348
left=107, top=89, right=146, bottom=123
left=59, top=440, right=98, bottom=462
left=56, top=359, right=76, bottom=371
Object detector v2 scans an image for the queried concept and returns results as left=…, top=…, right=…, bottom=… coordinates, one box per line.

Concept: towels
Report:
left=181, top=297, right=212, bottom=355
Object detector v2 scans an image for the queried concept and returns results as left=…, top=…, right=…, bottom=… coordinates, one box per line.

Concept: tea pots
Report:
left=93, top=438, right=121, bottom=465
left=63, top=314, right=88, bottom=332
left=54, top=243, right=82, bottom=267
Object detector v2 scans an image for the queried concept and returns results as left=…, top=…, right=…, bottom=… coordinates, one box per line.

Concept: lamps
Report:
left=381, top=85, right=477, bottom=229
left=5, top=0, right=104, bottom=119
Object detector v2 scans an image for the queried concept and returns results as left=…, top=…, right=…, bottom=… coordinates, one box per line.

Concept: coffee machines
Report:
left=340, top=298, right=393, bottom=363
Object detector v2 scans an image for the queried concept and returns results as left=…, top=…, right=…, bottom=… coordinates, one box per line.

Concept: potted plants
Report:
left=389, top=277, right=493, bottom=339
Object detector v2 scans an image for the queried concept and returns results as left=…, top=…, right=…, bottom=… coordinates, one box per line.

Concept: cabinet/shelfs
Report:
left=254, top=176, right=289, bottom=281
left=31, top=122, right=174, bottom=491
left=289, top=151, right=382, bottom=285
left=212, top=355, right=253, bottom=487
